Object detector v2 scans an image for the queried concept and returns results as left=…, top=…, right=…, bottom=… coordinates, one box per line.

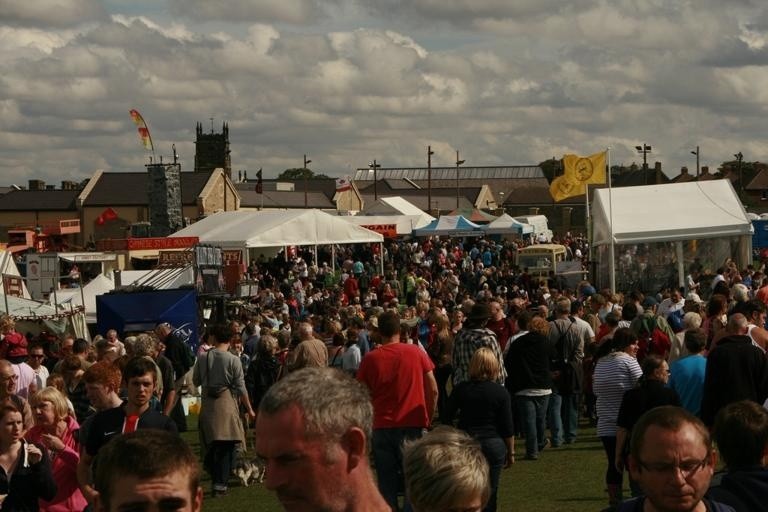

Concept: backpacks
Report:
left=550, top=319, right=576, bottom=383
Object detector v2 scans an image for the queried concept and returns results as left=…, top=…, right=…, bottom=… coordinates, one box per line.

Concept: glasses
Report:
left=635, top=452, right=710, bottom=479
left=658, top=368, right=672, bottom=376
left=30, top=354, right=44, bottom=359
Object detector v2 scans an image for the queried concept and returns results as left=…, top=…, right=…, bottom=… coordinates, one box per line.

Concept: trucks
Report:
left=516, top=243, right=568, bottom=294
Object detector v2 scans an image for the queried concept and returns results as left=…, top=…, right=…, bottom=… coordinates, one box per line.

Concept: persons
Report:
left=2, top=228, right=768, bottom=511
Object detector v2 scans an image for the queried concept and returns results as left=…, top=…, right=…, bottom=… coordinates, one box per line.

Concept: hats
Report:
left=641, top=296, right=660, bottom=306
left=468, top=304, right=490, bottom=321
left=685, top=292, right=704, bottom=303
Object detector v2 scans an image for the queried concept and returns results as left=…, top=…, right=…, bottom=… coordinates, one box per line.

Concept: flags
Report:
left=562, top=151, right=606, bottom=185
left=255, top=169, right=264, bottom=193
left=538, top=156, right=585, bottom=202
left=130, top=109, right=153, bottom=152
left=334, top=174, right=351, bottom=192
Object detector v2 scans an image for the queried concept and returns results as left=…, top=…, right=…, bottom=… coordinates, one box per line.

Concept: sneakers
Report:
left=523, top=435, right=576, bottom=461
left=211, top=489, right=228, bottom=499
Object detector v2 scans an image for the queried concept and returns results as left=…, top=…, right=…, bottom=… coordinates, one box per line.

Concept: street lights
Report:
left=427, top=146, right=436, bottom=209
left=302, top=154, right=311, bottom=206
left=691, top=145, right=700, bottom=182
left=635, top=143, right=652, bottom=184
left=733, top=151, right=745, bottom=204
left=455, top=149, right=465, bottom=208
left=367, top=159, right=381, bottom=202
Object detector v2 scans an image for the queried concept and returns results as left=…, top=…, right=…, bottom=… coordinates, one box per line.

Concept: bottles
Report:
left=421, top=427, right=428, bottom=437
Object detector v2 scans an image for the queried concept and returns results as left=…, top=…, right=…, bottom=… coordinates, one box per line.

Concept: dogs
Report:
left=231, top=449, right=267, bottom=488
